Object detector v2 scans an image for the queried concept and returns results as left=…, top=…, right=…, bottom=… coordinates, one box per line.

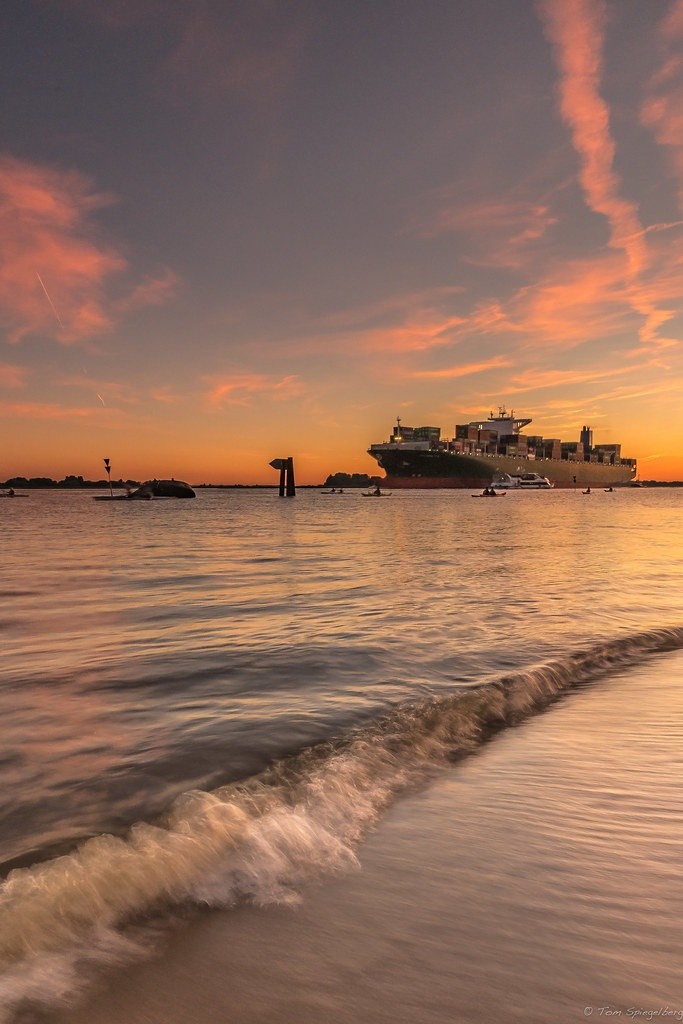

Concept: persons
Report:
left=483, top=487, right=496, bottom=495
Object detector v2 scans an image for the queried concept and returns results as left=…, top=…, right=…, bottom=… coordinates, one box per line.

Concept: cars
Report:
left=631, top=483, right=641, bottom=487
left=125, top=477, right=197, bottom=498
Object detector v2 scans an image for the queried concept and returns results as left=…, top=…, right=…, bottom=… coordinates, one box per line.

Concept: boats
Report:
left=604, top=489, right=616, bottom=492
left=0, top=493, right=29, bottom=498
left=470, top=492, right=506, bottom=497
left=321, top=491, right=345, bottom=494
left=490, top=473, right=555, bottom=489
left=361, top=492, right=393, bottom=496
left=367, top=407, right=637, bottom=488
left=582, top=491, right=590, bottom=494
